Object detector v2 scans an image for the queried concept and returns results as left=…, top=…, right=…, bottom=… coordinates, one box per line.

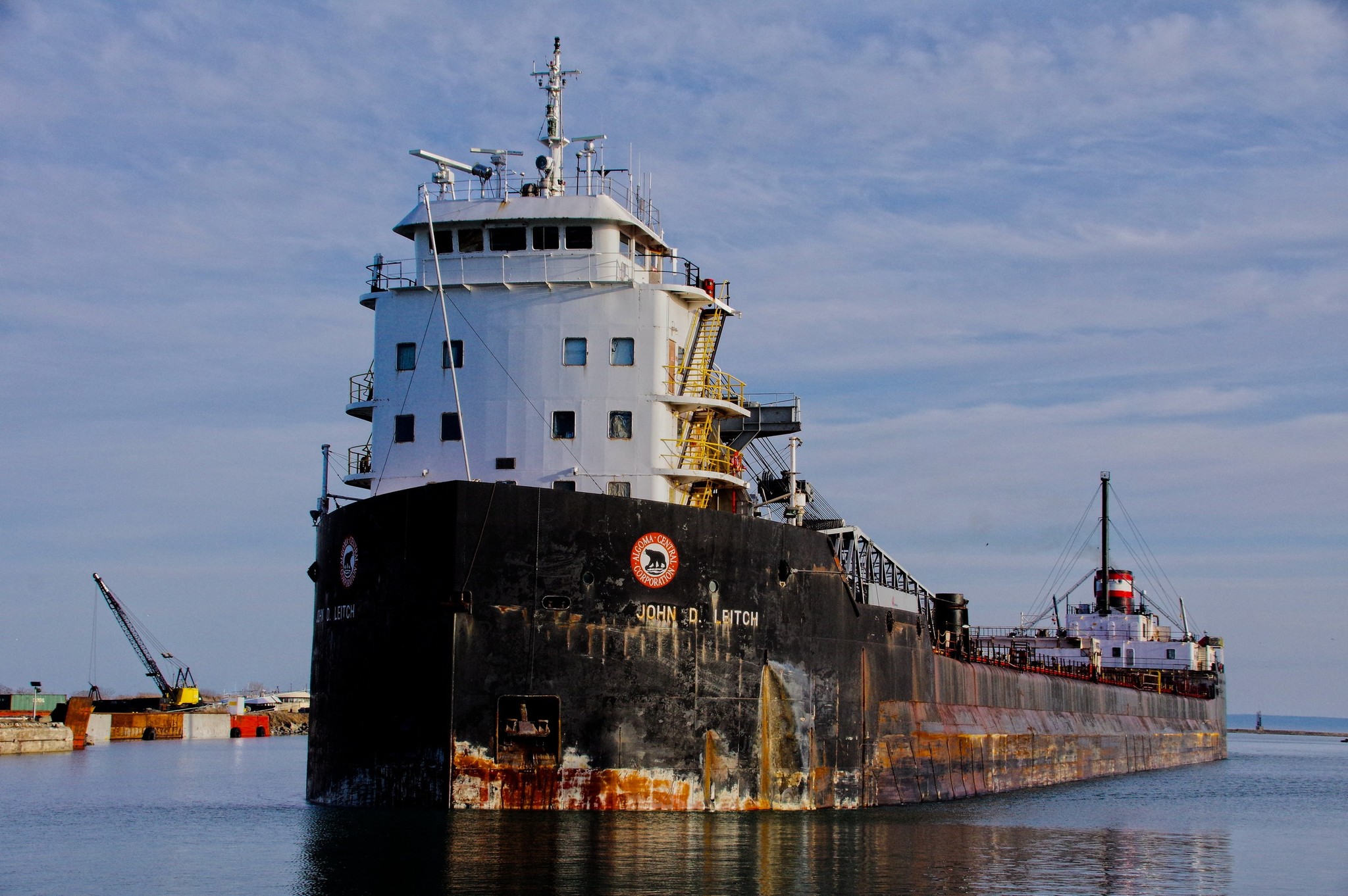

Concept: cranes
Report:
left=87, top=572, right=204, bottom=709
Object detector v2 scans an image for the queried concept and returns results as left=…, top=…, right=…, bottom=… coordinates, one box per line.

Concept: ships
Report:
left=300, top=35, right=1225, bottom=812
